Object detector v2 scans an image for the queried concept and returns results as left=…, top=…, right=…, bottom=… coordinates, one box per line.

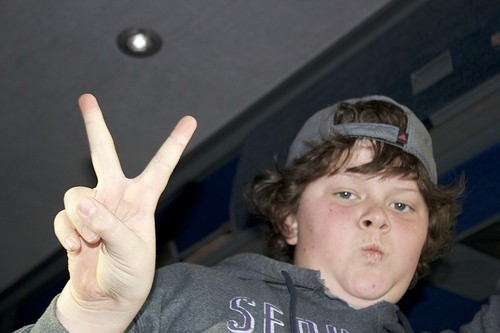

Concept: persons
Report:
left=14, top=92, right=500, bottom=333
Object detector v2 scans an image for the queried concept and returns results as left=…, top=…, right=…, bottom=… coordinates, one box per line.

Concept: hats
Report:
left=284, top=95, right=437, bottom=184
